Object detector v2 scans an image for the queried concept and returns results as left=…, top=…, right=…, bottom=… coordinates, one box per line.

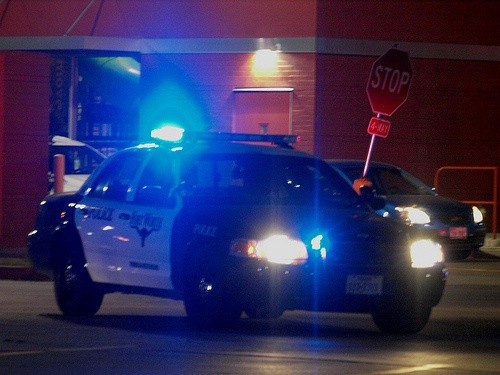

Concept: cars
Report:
left=321, top=158, right=487, bottom=261
left=26, top=132, right=448, bottom=337
left=48, top=135, right=109, bottom=199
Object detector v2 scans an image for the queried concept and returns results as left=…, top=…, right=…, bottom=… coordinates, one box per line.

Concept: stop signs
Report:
left=366, top=48, right=415, bottom=118
